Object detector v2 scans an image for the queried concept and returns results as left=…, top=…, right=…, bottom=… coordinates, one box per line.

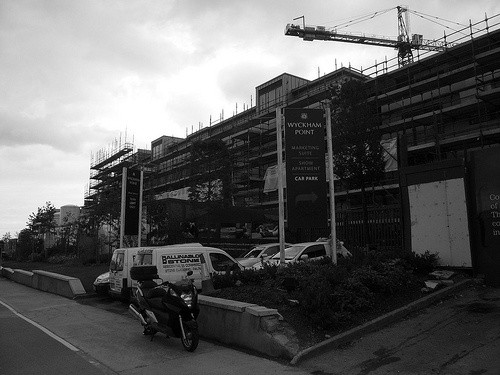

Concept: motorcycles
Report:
left=127, top=264, right=201, bottom=353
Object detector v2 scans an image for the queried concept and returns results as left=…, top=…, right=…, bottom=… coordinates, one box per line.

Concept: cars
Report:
left=234, top=243, right=292, bottom=268
left=247, top=240, right=352, bottom=270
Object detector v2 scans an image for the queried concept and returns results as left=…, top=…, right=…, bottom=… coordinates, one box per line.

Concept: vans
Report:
left=108, top=246, right=247, bottom=305
left=92, top=243, right=204, bottom=299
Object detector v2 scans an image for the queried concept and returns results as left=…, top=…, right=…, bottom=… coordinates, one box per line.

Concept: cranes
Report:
left=284, top=5, right=486, bottom=65
